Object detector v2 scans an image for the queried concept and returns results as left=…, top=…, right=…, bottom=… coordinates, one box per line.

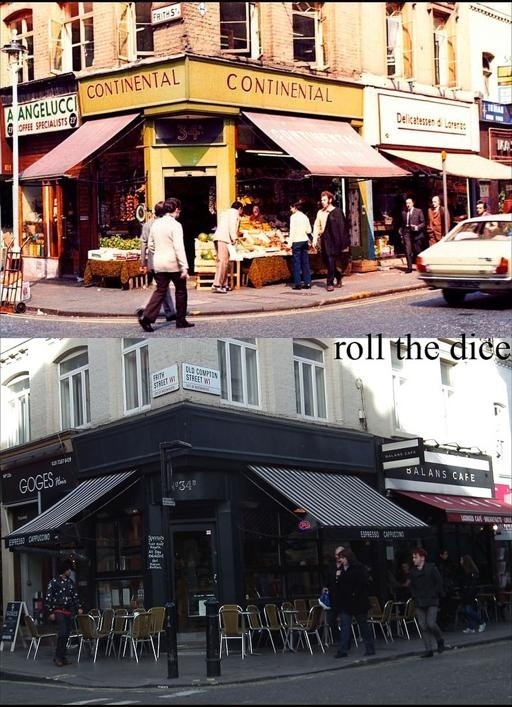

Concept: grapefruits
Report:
left=197, top=232, right=213, bottom=242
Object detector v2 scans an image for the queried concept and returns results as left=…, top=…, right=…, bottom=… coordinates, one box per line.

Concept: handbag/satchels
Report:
left=43, top=608, right=71, bottom=635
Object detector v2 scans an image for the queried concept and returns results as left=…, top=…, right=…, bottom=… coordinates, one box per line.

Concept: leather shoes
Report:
left=292, top=277, right=342, bottom=291
left=139, top=311, right=195, bottom=332
left=405, top=269, right=412, bottom=273
left=212, top=285, right=232, bottom=294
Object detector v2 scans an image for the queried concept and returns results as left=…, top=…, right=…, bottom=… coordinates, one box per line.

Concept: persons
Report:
left=210, top=200, right=243, bottom=292
left=287, top=199, right=314, bottom=290
left=137, top=199, right=195, bottom=332
left=317, top=544, right=487, bottom=659
left=135, top=201, right=188, bottom=323
left=426, top=195, right=451, bottom=248
left=399, top=198, right=426, bottom=273
left=312, top=190, right=350, bottom=292
left=45, top=563, right=83, bottom=666
left=470, top=199, right=492, bottom=236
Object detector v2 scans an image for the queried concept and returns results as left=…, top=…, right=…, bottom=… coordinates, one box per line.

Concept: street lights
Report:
left=1, top=31, right=30, bottom=289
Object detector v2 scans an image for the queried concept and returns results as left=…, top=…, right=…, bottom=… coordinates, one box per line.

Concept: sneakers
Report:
left=334, top=651, right=347, bottom=657
left=363, top=651, right=374, bottom=656
left=421, top=638, right=443, bottom=658
left=463, top=622, right=486, bottom=633
left=53, top=658, right=63, bottom=667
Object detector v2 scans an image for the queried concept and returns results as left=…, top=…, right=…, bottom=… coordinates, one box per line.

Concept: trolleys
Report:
left=4, top=234, right=35, bottom=313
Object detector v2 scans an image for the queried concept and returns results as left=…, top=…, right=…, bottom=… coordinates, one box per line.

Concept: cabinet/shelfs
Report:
left=195, top=227, right=347, bottom=291
left=85, top=236, right=149, bottom=289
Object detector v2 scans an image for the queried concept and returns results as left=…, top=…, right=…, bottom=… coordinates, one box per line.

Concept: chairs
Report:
left=23, top=595, right=511, bottom=663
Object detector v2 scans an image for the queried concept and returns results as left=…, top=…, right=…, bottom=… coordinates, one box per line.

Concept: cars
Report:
left=418, top=215, right=511, bottom=307
